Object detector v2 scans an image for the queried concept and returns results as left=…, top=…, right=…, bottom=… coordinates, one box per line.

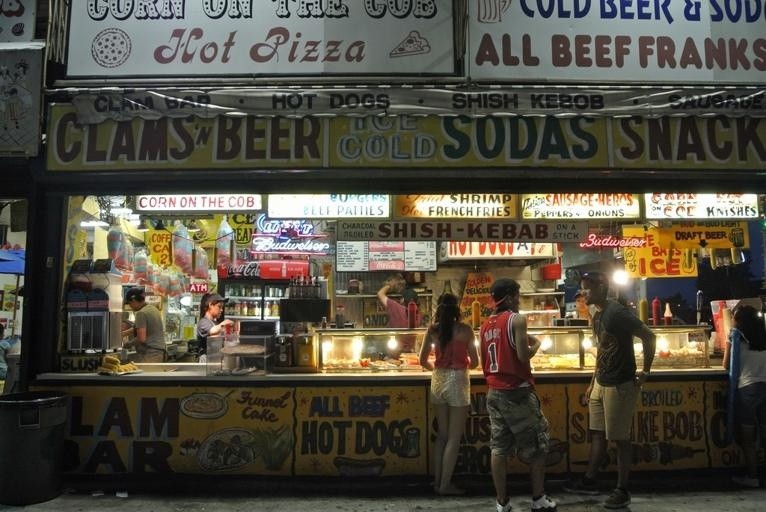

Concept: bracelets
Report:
left=642, top=370, right=651, bottom=374
left=386, top=283, right=392, bottom=288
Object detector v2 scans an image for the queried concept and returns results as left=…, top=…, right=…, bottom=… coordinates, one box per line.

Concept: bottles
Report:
left=321, top=317, right=327, bottom=329
left=639, top=296, right=649, bottom=325
left=224, top=274, right=322, bottom=318
left=471, top=295, right=482, bottom=331
left=663, top=302, right=673, bottom=325
left=407, top=297, right=417, bottom=328
left=651, top=295, right=662, bottom=325
left=335, top=304, right=346, bottom=328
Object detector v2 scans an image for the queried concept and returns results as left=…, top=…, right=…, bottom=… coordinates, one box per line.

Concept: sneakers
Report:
left=604, top=489, right=632, bottom=508
left=530, top=495, right=557, bottom=511
left=495, top=496, right=511, bottom=512
left=733, top=475, right=759, bottom=488
left=561, top=477, right=600, bottom=495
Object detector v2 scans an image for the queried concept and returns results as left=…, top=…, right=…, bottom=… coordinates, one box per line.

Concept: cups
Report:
left=728, top=228, right=744, bottom=247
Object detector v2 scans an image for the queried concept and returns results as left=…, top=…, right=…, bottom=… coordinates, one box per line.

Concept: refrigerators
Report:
left=217, top=260, right=309, bottom=335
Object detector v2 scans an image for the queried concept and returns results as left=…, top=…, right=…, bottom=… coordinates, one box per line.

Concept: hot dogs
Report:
left=333, top=456, right=386, bottom=478
left=517, top=439, right=566, bottom=465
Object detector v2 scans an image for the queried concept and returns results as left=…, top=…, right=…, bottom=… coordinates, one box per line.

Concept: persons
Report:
left=120, top=288, right=166, bottom=364
left=559, top=271, right=660, bottom=510
left=418, top=292, right=481, bottom=495
left=556, top=265, right=584, bottom=317
left=565, top=286, right=596, bottom=326
left=196, top=291, right=234, bottom=362
left=477, top=277, right=560, bottom=511
left=372, top=276, right=424, bottom=360
left=721, top=304, right=766, bottom=487
left=0, top=323, right=12, bottom=381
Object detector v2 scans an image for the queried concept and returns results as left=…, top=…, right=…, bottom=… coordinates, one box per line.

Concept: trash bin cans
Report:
left=0, top=390, right=71, bottom=507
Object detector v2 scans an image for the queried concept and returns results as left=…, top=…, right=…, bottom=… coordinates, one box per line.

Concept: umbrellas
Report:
left=0, top=248, right=26, bottom=343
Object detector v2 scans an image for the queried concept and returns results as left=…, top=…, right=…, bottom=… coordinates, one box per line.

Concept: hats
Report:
left=204, top=294, right=228, bottom=304
left=400, top=289, right=418, bottom=302
left=124, top=289, right=144, bottom=303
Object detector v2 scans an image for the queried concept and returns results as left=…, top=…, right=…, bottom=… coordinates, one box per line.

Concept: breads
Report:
left=100, top=356, right=137, bottom=373
left=636, top=348, right=706, bottom=370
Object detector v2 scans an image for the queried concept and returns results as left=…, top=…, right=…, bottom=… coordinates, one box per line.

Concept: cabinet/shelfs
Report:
left=216, top=278, right=293, bottom=373
left=310, top=324, right=714, bottom=374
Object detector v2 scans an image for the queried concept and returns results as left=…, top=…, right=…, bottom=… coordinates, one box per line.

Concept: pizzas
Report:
left=218, top=341, right=266, bottom=355
left=185, top=393, right=225, bottom=416
